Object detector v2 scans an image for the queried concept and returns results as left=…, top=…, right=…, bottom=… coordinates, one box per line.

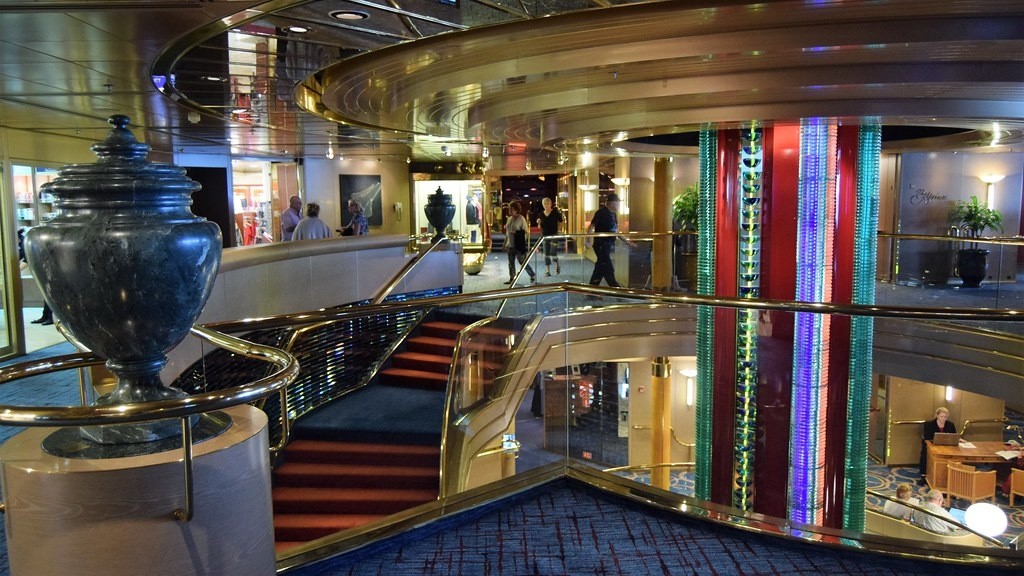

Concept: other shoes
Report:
left=554, top=265, right=561, bottom=275
left=503, top=279, right=512, bottom=284
left=31, top=317, right=53, bottom=325
left=530, top=274, right=535, bottom=283
left=544, top=272, right=551, bottom=277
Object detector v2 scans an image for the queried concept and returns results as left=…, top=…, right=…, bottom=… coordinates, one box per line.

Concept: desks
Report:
left=923, top=438, right=1024, bottom=496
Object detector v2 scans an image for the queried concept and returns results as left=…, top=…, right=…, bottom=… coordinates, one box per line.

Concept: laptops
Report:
left=930, top=433, right=960, bottom=446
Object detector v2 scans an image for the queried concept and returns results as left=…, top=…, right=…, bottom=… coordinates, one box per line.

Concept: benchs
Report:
left=865, top=506, right=984, bottom=545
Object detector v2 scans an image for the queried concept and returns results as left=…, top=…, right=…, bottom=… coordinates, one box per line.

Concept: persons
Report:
left=536, top=197, right=564, bottom=277
left=586, top=193, right=638, bottom=302
left=280, top=196, right=304, bottom=241
left=916, top=407, right=968, bottom=487
left=291, top=202, right=332, bottom=241
left=642, top=220, right=688, bottom=293
left=18, top=228, right=28, bottom=270
left=1000, top=447, right=1024, bottom=499
left=884, top=482, right=912, bottom=521
left=340, top=199, right=368, bottom=236
left=913, top=489, right=964, bottom=533
left=502, top=201, right=536, bottom=284
left=465, top=193, right=483, bottom=242
left=31, top=191, right=54, bottom=325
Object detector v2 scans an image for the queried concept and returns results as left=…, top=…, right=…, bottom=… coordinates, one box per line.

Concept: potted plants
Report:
left=672, top=180, right=700, bottom=292
left=944, top=193, right=1006, bottom=289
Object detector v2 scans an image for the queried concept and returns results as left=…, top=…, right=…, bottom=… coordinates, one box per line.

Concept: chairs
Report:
left=1009, top=466, right=1024, bottom=509
left=947, top=458, right=997, bottom=508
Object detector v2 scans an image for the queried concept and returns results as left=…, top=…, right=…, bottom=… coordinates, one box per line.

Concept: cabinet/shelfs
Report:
left=13, top=173, right=59, bottom=307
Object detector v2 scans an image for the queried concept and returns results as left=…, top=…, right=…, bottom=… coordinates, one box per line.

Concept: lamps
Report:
left=610, top=177, right=630, bottom=214
left=578, top=184, right=598, bottom=211
left=945, top=386, right=953, bottom=401
left=326, top=142, right=335, bottom=159
left=977, top=174, right=1006, bottom=210
left=678, top=369, right=697, bottom=408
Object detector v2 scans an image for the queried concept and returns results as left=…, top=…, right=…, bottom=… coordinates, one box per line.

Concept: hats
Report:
left=606, top=194, right=622, bottom=202
